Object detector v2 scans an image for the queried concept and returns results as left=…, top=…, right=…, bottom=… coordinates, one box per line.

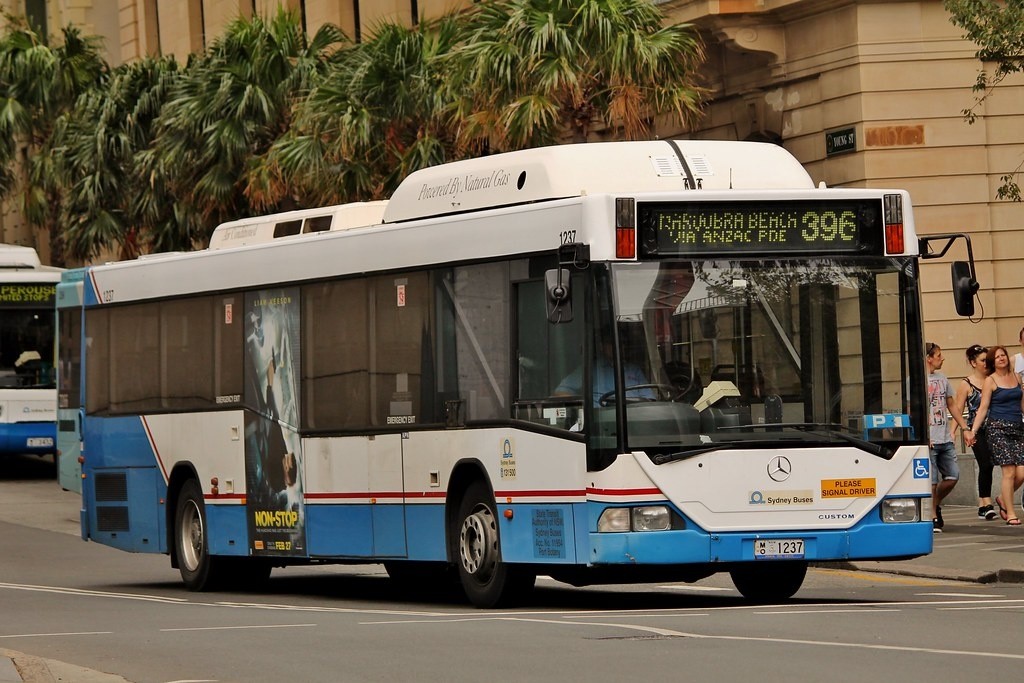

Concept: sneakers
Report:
left=978, top=505, right=999, bottom=521
left=933, top=504, right=944, bottom=533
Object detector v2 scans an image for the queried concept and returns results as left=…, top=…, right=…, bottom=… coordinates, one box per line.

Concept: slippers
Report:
left=996, top=497, right=1008, bottom=520
left=1006, top=517, right=1021, bottom=525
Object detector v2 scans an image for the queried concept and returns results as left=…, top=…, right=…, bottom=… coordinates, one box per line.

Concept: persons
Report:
left=970, top=345, right=1024, bottom=524
left=906, top=342, right=973, bottom=533
left=950, top=345, right=1000, bottom=521
left=552, top=338, right=657, bottom=409
left=1008, top=326, right=1024, bottom=423
left=263, top=363, right=298, bottom=492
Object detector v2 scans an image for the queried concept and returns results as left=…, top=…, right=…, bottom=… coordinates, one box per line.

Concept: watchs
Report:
left=962, top=427, right=971, bottom=432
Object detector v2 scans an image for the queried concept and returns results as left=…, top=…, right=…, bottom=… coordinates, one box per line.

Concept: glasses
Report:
left=974, top=346, right=985, bottom=351
left=928, top=343, right=936, bottom=355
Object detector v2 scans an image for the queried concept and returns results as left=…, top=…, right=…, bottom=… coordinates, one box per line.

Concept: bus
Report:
left=55, top=266, right=89, bottom=497
left=82, top=141, right=984, bottom=609
left=0, top=243, right=70, bottom=464
